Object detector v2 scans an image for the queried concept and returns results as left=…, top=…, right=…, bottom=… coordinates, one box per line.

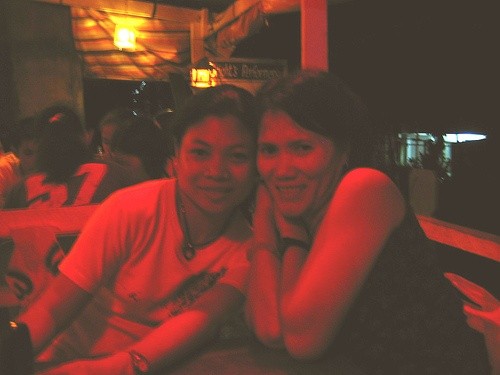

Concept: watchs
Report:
left=123, top=348, right=151, bottom=375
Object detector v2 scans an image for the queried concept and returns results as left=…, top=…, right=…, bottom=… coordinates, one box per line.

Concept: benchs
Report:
left=1, top=202, right=500, bottom=375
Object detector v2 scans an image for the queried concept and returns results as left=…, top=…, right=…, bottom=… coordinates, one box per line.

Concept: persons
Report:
left=242, top=67, right=490, bottom=375
left=15, top=85, right=259, bottom=375
left=1, top=100, right=178, bottom=207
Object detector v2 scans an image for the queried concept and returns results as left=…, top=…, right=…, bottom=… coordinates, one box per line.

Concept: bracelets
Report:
left=246, top=242, right=280, bottom=260
left=280, top=236, right=310, bottom=256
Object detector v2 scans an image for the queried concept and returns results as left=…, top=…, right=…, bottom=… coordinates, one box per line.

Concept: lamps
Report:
left=190, top=56, right=223, bottom=89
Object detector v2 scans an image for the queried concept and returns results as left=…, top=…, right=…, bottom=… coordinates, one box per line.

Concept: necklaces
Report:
left=178, top=195, right=231, bottom=260
